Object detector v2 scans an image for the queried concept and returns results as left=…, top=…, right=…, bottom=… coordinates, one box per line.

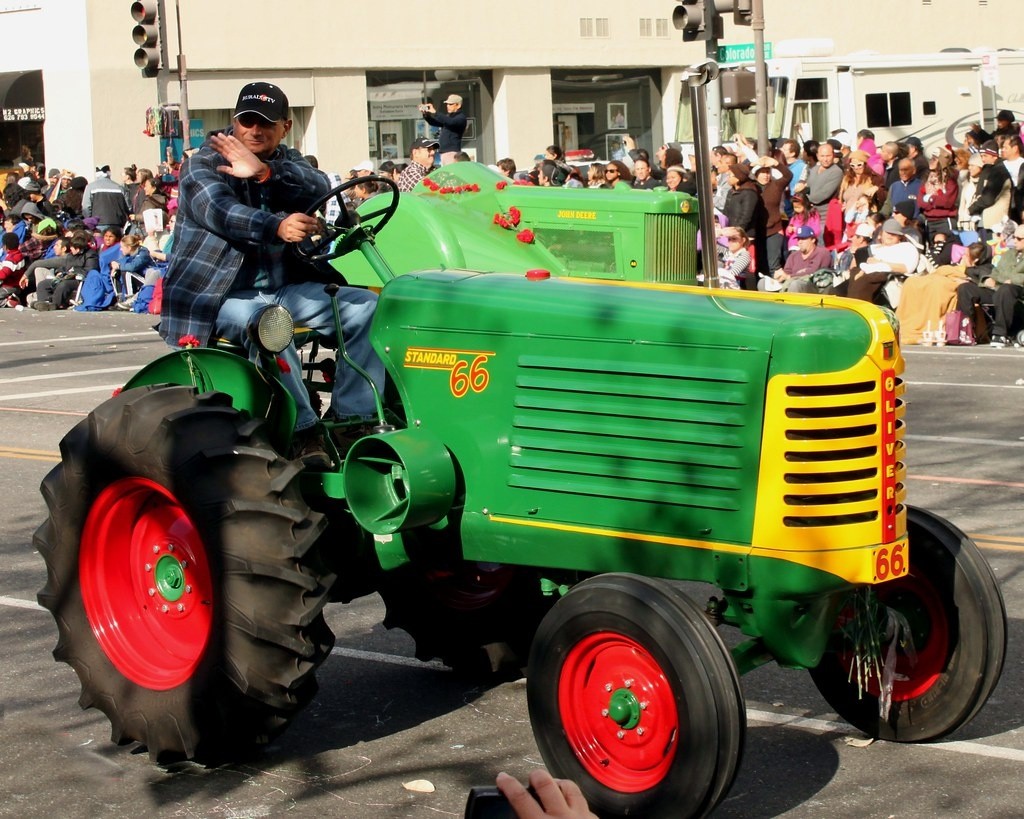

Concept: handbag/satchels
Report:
left=945, top=310, right=975, bottom=345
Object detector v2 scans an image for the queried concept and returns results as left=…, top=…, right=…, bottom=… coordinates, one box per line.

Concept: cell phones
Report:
left=419, top=105, right=428, bottom=111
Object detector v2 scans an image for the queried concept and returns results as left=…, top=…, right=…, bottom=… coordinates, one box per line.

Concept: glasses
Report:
left=605, top=169, right=619, bottom=173
left=1012, top=235, right=1024, bottom=241
left=850, top=162, right=864, bottom=168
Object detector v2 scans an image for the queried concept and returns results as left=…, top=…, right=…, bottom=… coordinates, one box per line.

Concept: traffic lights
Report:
left=130, top=0, right=166, bottom=80
left=672, top=0, right=723, bottom=40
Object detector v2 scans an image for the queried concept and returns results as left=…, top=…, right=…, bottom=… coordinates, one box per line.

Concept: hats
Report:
left=978, top=139, right=999, bottom=156
left=666, top=142, right=682, bottom=152
left=232, top=82, right=289, bottom=122
left=26, top=181, right=41, bottom=194
left=828, top=132, right=852, bottom=146
left=931, top=147, right=939, bottom=157
left=895, top=199, right=915, bottom=221
left=666, top=165, right=686, bottom=173
left=60, top=169, right=75, bottom=180
left=83, top=216, right=100, bottom=230
left=883, top=218, right=904, bottom=236
left=844, top=222, right=873, bottom=238
left=443, top=94, right=463, bottom=105
left=994, top=110, right=1015, bottom=122
left=353, top=160, right=374, bottom=172
left=793, top=226, right=815, bottom=239
left=849, top=150, right=871, bottom=163
left=906, top=137, right=921, bottom=147
left=729, top=164, right=749, bottom=183
left=409, top=137, right=440, bottom=152
left=542, top=159, right=571, bottom=187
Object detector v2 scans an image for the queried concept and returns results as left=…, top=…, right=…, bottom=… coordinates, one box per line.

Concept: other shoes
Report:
left=5, top=293, right=138, bottom=311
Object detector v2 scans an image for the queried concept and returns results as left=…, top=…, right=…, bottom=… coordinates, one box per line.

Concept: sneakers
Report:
left=990, top=334, right=1008, bottom=347
left=299, top=423, right=331, bottom=472
left=329, top=425, right=373, bottom=459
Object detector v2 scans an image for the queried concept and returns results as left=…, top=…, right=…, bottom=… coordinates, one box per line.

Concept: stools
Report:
left=972, top=303, right=1020, bottom=345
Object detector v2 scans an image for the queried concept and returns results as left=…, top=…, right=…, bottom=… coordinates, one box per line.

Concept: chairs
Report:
left=167, top=235, right=312, bottom=351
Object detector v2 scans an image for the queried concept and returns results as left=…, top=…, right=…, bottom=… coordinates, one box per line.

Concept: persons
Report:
left=419, top=93, right=467, bottom=165
left=305, top=138, right=471, bottom=227
left=695, top=110, right=1024, bottom=346
left=151, top=81, right=389, bottom=460
left=0, top=144, right=202, bottom=315
left=495, top=142, right=698, bottom=197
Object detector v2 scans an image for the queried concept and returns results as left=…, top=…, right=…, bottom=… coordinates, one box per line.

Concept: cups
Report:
left=14, top=304, right=24, bottom=312
left=934, top=330, right=946, bottom=346
left=923, top=330, right=933, bottom=346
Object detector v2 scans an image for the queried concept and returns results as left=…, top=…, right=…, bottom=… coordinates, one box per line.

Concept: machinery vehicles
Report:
left=32, top=160, right=1011, bottom=819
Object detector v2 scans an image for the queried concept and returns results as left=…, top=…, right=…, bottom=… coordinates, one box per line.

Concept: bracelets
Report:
left=257, top=168, right=271, bottom=184
